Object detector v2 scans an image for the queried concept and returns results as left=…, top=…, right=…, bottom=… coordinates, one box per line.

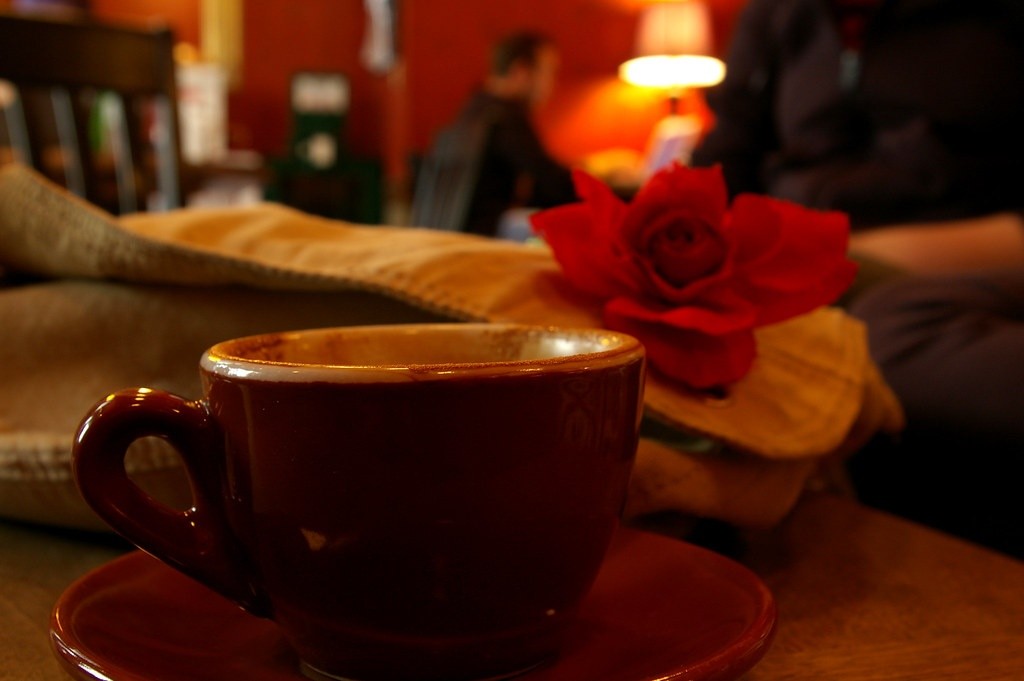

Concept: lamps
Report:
left=618, top=0, right=727, bottom=116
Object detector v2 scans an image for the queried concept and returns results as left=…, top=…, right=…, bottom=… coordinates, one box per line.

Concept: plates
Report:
left=48, top=518, right=775, bottom=681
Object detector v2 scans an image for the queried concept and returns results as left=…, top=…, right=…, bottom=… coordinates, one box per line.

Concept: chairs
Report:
left=0, top=14, right=184, bottom=217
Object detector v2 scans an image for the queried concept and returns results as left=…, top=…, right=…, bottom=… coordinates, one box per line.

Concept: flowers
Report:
left=525, top=161, right=861, bottom=390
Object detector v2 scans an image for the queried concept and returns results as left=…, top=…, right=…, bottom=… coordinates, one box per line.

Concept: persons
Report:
left=695, top=0, right=1024, bottom=563
left=449, top=31, right=583, bottom=238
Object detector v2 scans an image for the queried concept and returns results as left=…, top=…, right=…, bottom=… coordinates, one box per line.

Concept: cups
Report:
left=72, top=325, right=648, bottom=681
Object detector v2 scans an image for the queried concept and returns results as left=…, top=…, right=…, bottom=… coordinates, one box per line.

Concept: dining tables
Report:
left=0, top=488, right=1023, bottom=681
